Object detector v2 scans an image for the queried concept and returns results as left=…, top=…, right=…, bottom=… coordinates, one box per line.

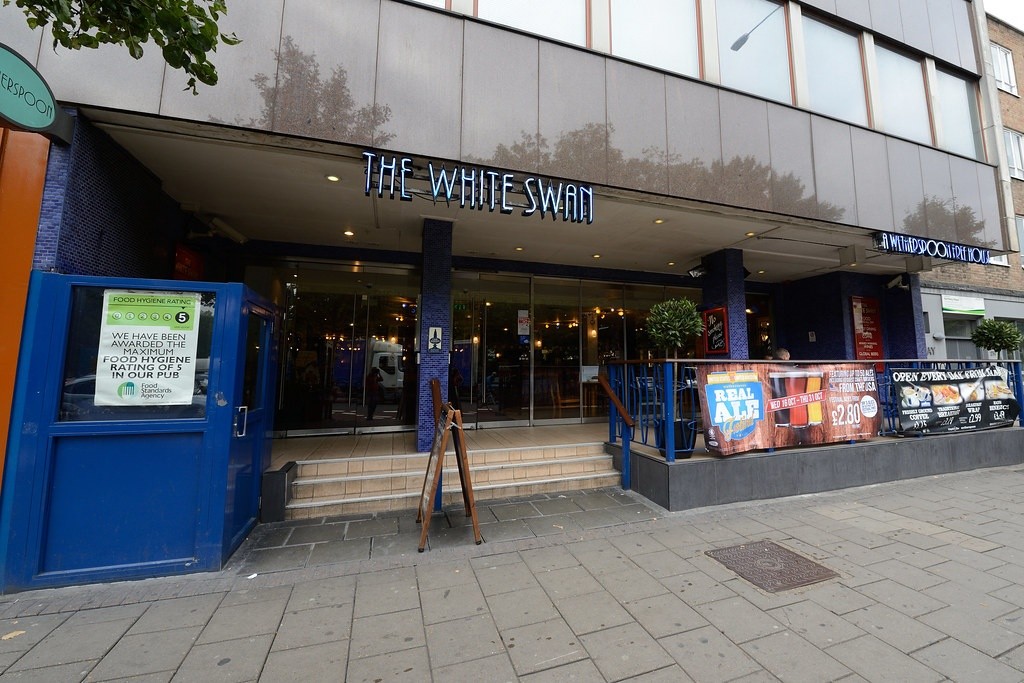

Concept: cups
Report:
left=769, top=372, right=790, bottom=427
left=903, top=389, right=919, bottom=406
left=920, top=387, right=931, bottom=401
left=806, top=373, right=824, bottom=425
left=785, top=372, right=809, bottom=428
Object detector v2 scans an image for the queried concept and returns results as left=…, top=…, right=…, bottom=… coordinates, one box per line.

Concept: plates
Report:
left=901, top=398, right=920, bottom=408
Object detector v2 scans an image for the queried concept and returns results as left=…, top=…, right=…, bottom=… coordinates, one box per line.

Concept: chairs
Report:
left=875, top=375, right=891, bottom=437
left=636, top=374, right=666, bottom=426
left=548, top=373, right=580, bottom=419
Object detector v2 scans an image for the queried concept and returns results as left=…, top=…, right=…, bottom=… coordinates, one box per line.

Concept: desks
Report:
left=583, top=380, right=605, bottom=417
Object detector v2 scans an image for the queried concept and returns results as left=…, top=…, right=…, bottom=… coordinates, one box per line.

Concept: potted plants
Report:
left=644, top=299, right=703, bottom=458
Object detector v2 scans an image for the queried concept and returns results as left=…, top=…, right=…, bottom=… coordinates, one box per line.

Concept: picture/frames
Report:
left=704, top=307, right=729, bottom=354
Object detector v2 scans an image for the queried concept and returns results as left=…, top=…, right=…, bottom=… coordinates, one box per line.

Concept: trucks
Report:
left=369, top=338, right=404, bottom=391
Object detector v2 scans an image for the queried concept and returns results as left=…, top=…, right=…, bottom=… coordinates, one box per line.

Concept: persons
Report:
left=486, top=372, right=496, bottom=393
left=762, top=349, right=796, bottom=366
left=453, top=368, right=464, bottom=411
left=366, top=367, right=383, bottom=421
